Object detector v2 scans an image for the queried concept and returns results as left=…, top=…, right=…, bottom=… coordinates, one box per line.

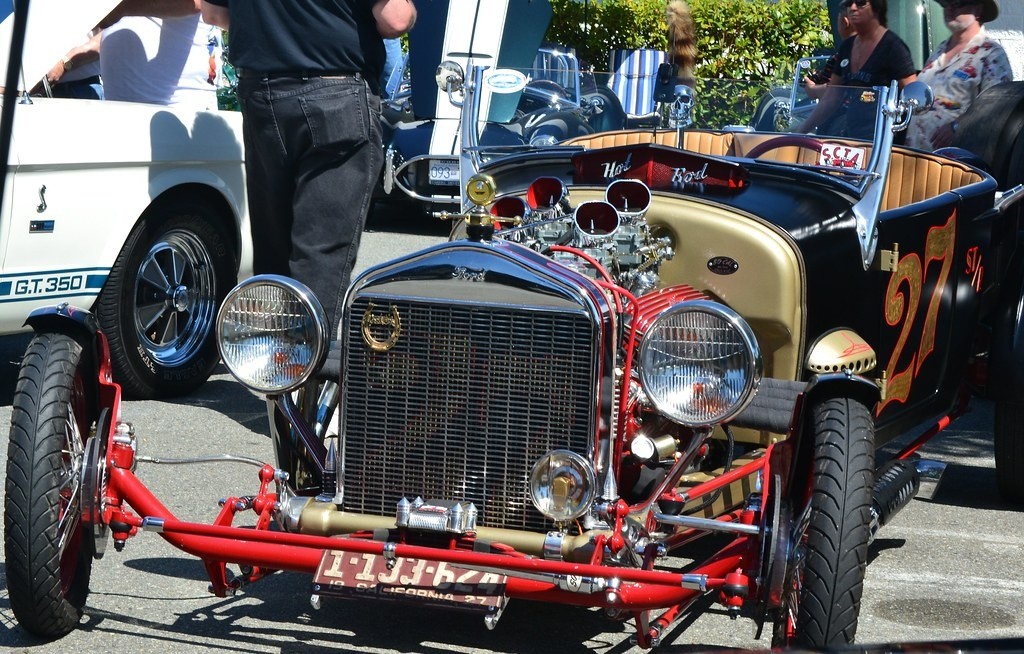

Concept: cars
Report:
left=0, top=0, right=631, bottom=392
left=2, top=50, right=1024, bottom=654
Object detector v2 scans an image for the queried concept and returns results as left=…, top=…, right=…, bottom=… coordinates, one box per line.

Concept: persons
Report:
left=794, top=0, right=916, bottom=141
left=0, top=29, right=105, bottom=101
left=904, top=0, right=1013, bottom=152
left=200, top=0, right=417, bottom=438
left=99, top=0, right=218, bottom=110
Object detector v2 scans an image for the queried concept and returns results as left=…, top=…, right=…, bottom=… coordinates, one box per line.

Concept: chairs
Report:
left=607, top=49, right=667, bottom=125
left=531, top=44, right=578, bottom=88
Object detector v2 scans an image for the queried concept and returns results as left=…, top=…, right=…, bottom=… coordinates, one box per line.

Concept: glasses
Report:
left=845, top=0, right=870, bottom=7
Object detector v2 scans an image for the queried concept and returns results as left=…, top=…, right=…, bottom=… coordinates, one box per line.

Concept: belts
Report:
left=239, top=67, right=355, bottom=78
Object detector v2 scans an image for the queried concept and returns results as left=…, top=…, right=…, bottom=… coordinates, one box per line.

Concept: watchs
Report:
left=951, top=120, right=959, bottom=133
left=62, top=56, right=73, bottom=72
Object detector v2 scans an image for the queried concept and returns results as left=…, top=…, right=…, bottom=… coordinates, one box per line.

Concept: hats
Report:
left=934, top=0, right=999, bottom=22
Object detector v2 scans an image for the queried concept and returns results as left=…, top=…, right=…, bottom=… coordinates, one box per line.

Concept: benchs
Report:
left=558, top=128, right=998, bottom=218
